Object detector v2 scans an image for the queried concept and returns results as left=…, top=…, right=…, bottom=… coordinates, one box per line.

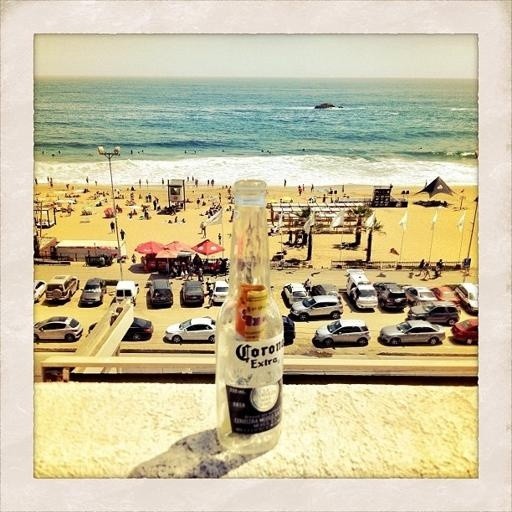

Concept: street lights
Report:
left=98, top=144, right=123, bottom=282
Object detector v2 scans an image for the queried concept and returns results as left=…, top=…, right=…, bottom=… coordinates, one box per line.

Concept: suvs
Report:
left=289, top=294, right=344, bottom=322
left=454, top=283, right=478, bottom=316
left=281, top=314, right=296, bottom=346
left=44, top=274, right=80, bottom=305
left=407, top=300, right=462, bottom=327
left=311, top=283, right=340, bottom=297
left=373, top=281, right=407, bottom=313
left=312, top=318, right=371, bottom=348
left=282, top=282, right=309, bottom=307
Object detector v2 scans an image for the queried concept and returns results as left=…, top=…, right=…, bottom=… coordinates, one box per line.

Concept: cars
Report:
left=451, top=317, right=478, bottom=345
left=35, top=280, right=47, bottom=303
left=164, top=315, right=216, bottom=345
left=35, top=315, right=83, bottom=342
left=403, top=285, right=438, bottom=307
left=87, top=313, right=154, bottom=342
left=380, top=319, right=447, bottom=347
left=179, top=280, right=204, bottom=307
left=430, top=286, right=461, bottom=308
left=78, top=277, right=104, bottom=306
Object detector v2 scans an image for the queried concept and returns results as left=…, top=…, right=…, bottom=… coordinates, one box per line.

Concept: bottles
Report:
left=217, top=178, right=284, bottom=458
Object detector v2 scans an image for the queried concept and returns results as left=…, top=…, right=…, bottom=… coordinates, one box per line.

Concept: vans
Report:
left=210, top=280, right=229, bottom=306
left=114, top=280, right=139, bottom=306
left=345, top=268, right=379, bottom=310
left=146, top=278, right=174, bottom=309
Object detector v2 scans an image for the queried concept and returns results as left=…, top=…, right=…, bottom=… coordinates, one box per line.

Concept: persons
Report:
left=129, top=253, right=227, bottom=306
left=305, top=278, right=312, bottom=292
left=407, top=307, right=415, bottom=320
left=33, top=174, right=236, bottom=241
left=265, top=179, right=472, bottom=254
left=33, top=148, right=311, bottom=157
left=417, top=258, right=444, bottom=280
